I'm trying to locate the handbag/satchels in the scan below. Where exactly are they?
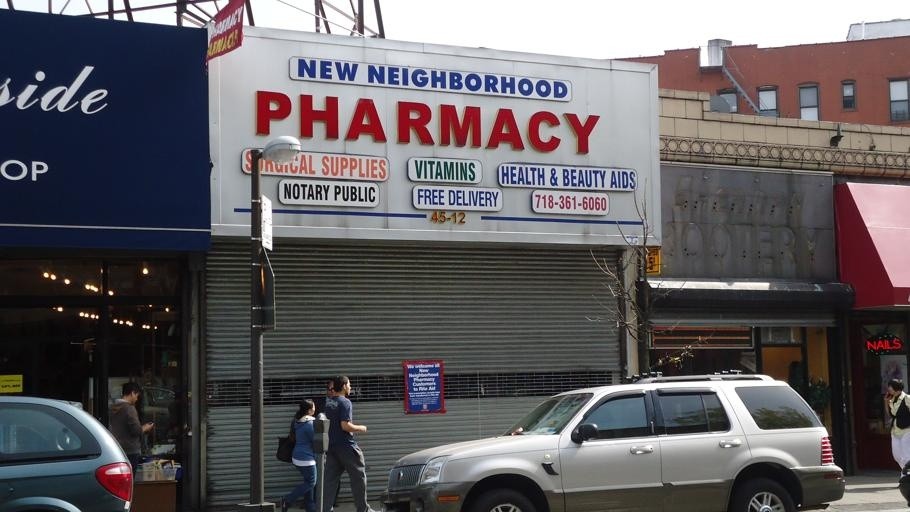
[277,437,295,463]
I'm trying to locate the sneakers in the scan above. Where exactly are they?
[280,496,289,512]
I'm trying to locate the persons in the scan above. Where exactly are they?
[883,378,910,486]
[314,379,342,508]
[106,379,156,486]
[323,375,377,512]
[279,397,318,511]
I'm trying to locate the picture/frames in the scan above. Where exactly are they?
[404,361,445,414]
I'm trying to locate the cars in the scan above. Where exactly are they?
[138,384,192,420]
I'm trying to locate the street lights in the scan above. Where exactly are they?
[235,134,304,511]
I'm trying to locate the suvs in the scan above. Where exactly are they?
[0,393,136,512]
[380,369,848,512]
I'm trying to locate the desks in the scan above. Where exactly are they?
[130,480,178,512]
